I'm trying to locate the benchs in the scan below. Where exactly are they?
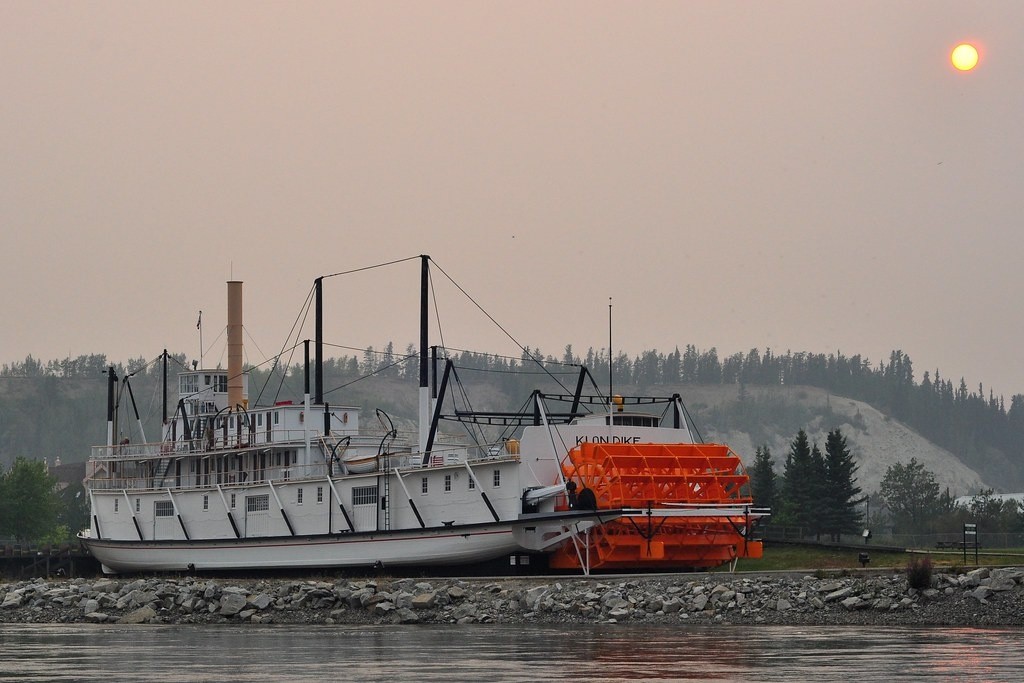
[935,545,981,548]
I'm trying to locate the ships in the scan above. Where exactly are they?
[77,251,776,578]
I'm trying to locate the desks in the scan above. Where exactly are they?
[936,541,979,550]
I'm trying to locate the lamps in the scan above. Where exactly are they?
[862,529,872,544]
[858,552,870,567]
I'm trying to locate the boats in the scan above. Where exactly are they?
[346,454,383,473]
